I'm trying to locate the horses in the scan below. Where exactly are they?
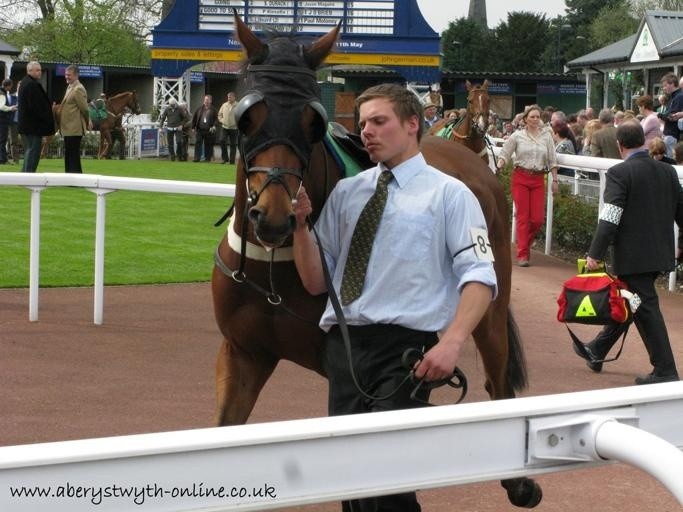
[40,89,142,160]
[427,78,490,166]
[210,5,543,509]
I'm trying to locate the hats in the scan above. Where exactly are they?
[422,100,436,109]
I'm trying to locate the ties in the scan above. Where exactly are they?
[339,168,395,307]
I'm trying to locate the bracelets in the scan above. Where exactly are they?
[552,180,557,183]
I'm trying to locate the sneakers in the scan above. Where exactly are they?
[169,156,236,164]
[516,258,530,267]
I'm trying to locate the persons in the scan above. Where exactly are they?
[18,59,56,173]
[571,118,682,385]
[158,94,216,163]
[216,92,240,166]
[0,78,22,166]
[394,72,682,202]
[290,81,498,511]
[55,64,93,189]
[496,102,558,268]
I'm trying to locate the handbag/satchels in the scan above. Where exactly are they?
[555,261,643,328]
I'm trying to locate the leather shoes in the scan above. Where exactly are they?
[571,340,603,373]
[634,370,680,385]
